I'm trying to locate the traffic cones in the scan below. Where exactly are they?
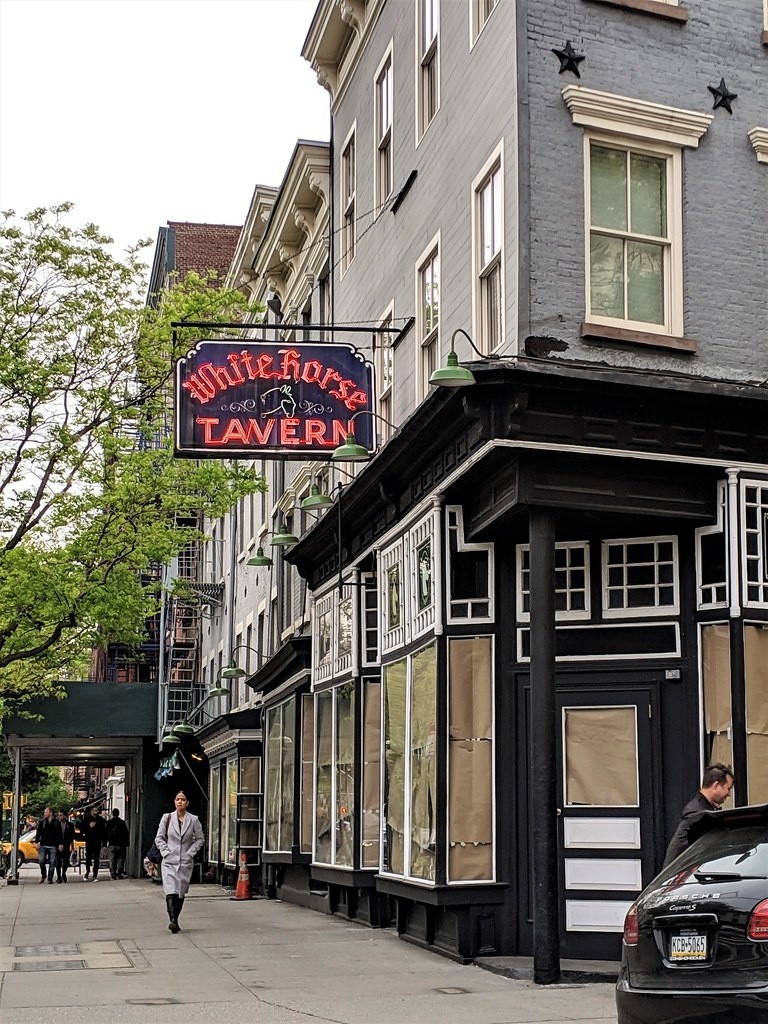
[228,855,260,900]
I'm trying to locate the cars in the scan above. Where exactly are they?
[0,828,90,868]
[613,802,768,1024]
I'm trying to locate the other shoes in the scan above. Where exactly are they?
[82,876,89,881]
[112,875,117,880]
[48,877,53,884]
[39,875,46,884]
[92,878,98,883]
[56,879,62,884]
[116,873,124,879]
[62,876,67,883]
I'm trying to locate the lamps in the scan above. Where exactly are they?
[331,410,398,460]
[429,328,491,387]
[246,532,279,565]
[269,507,319,545]
[222,645,271,679]
[301,466,354,509]
[162,720,198,743]
[173,707,216,733]
[210,666,251,696]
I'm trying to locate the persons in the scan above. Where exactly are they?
[664,762,735,867]
[102,809,130,879]
[154,791,205,934]
[36,807,60,883]
[81,807,106,882]
[27,824,35,831]
[53,809,75,883]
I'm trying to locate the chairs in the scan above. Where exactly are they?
[73,846,93,875]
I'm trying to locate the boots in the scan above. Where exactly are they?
[166,894,185,933]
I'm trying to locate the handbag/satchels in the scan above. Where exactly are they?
[148,814,172,865]
